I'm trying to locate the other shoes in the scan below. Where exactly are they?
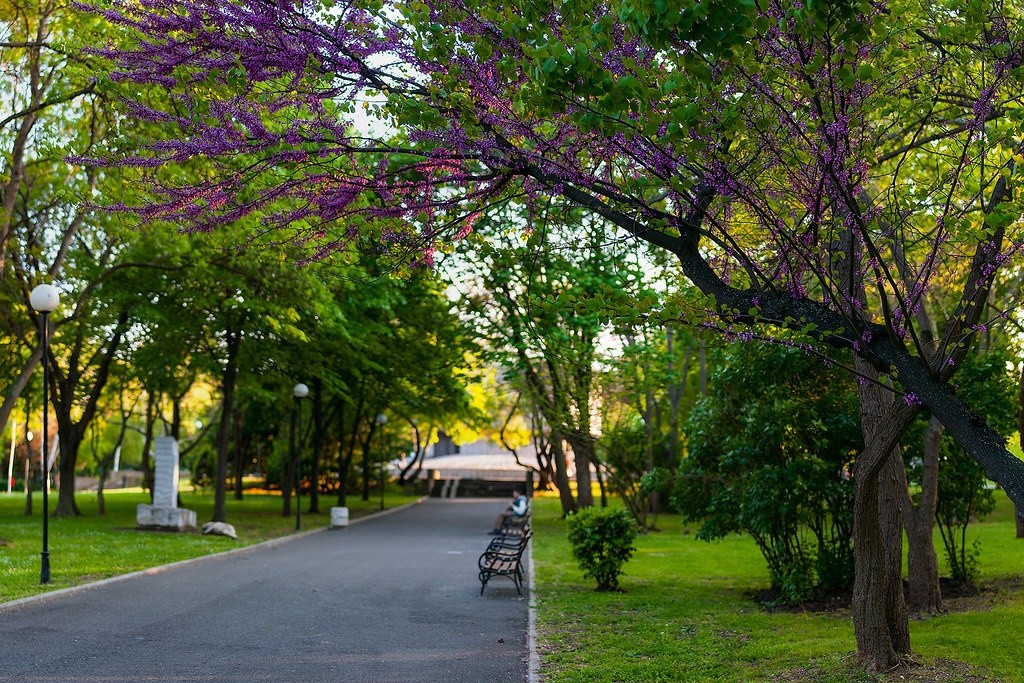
[488,528,502,535]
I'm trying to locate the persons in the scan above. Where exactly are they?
[487,486,527,535]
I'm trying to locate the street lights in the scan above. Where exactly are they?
[293,384,310,531]
[29,284,60,585]
[376,414,389,508]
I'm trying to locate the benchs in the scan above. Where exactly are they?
[477,496,534,596]
